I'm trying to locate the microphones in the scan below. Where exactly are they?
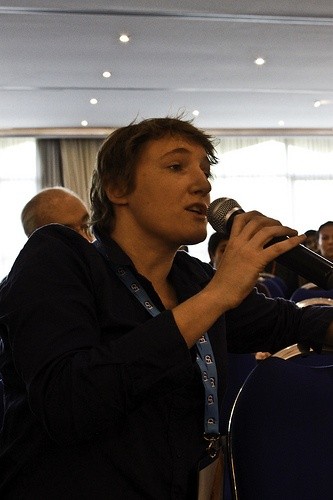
[206,198,333,293]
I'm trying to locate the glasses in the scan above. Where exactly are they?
[69,222,92,232]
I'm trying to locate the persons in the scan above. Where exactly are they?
[1,119,306,499]
[206,233,270,298]
[286,230,319,299]
[317,221,332,261]
[20,187,94,244]
